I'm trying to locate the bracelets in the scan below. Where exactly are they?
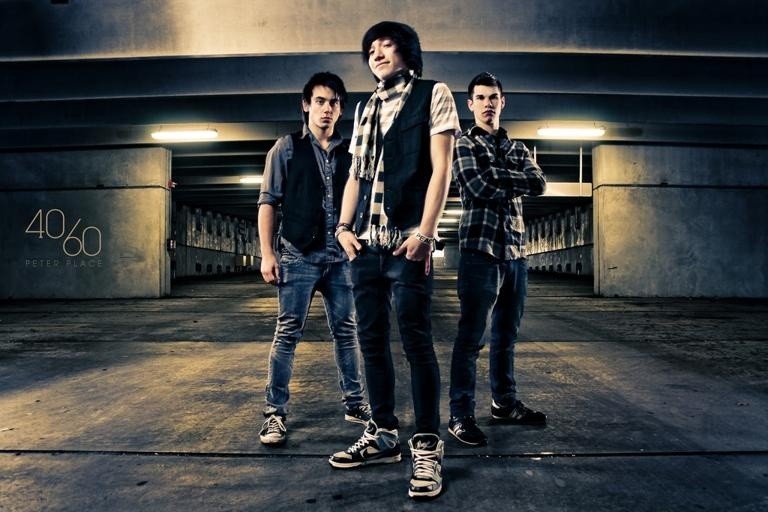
[414,232,435,247]
[334,222,352,238]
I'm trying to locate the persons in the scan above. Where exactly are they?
[324,21,464,504]
[256,70,374,448]
[445,71,550,446]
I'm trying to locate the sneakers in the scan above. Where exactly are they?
[328,417,402,469]
[490,398,548,427]
[344,400,374,427]
[258,403,288,445]
[406,432,445,499]
[447,414,488,447]
